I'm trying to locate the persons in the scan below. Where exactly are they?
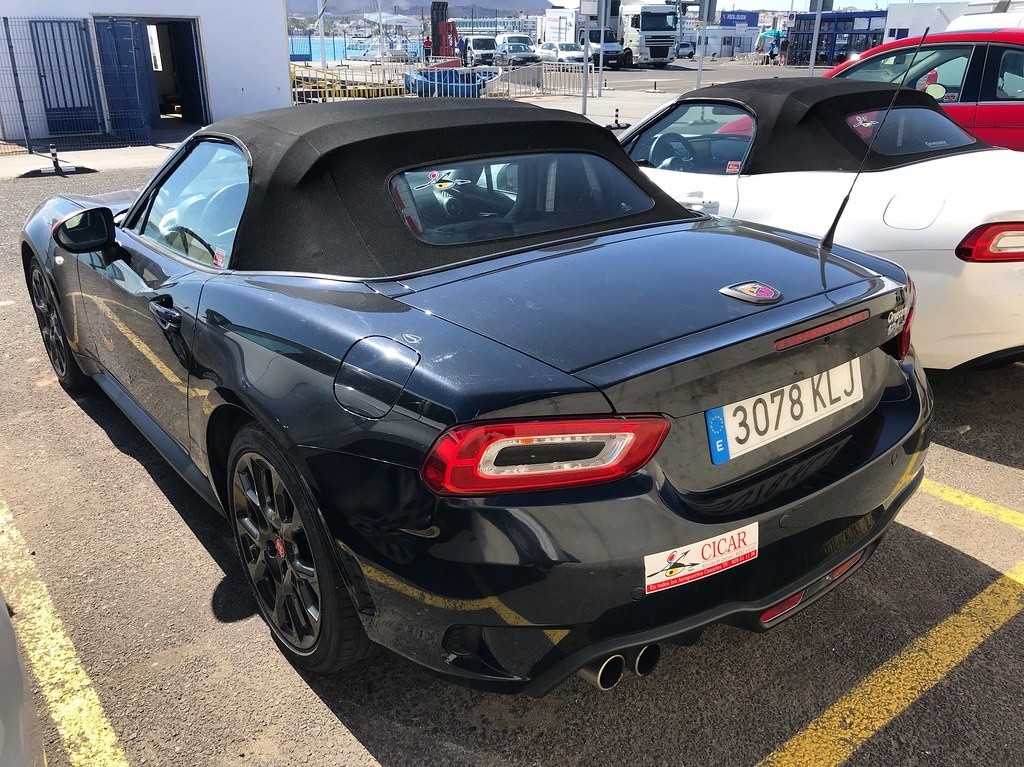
[768,33,791,66]
[422,35,433,66]
[456,35,467,70]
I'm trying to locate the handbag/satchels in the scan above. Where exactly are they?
[773,45,779,54]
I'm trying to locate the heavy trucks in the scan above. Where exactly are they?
[589,0,679,70]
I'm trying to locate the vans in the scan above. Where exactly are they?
[495,34,536,53]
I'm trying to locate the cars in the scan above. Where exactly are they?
[492,43,542,71]
[820,29,1024,153]
[536,41,594,73]
[679,42,694,58]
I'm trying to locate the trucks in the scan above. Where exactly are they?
[453,35,498,67]
[543,9,624,71]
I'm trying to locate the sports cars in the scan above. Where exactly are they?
[23,97,937,697]
[438,74,1024,375]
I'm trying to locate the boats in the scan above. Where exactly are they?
[345,35,422,62]
